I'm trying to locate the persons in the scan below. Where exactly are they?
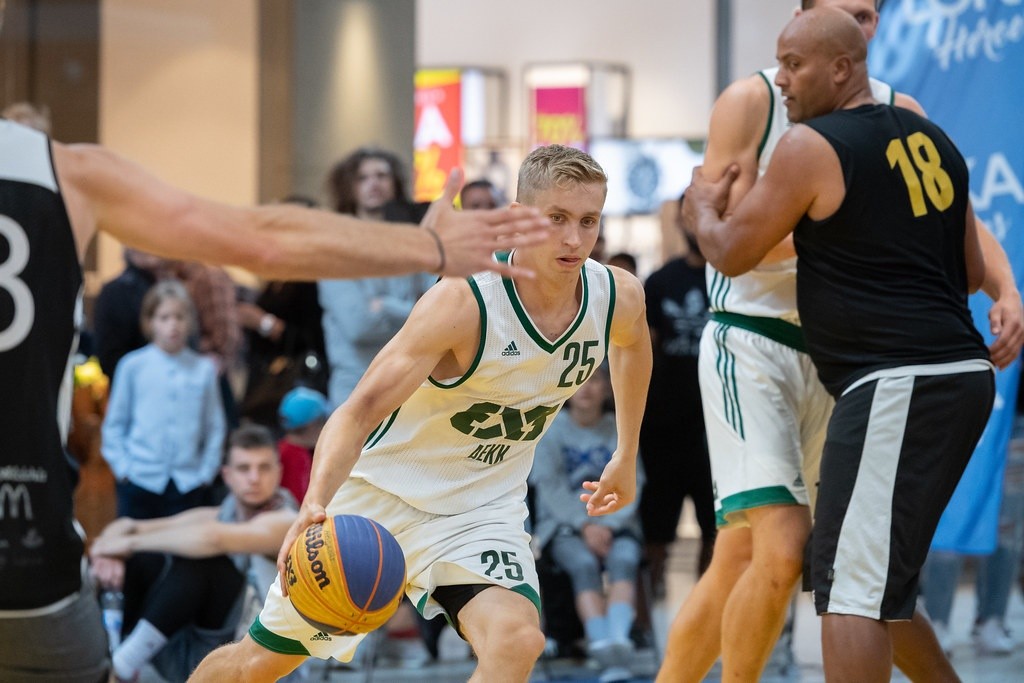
[0,0,1024,683]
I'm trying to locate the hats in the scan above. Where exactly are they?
[280,385,331,431]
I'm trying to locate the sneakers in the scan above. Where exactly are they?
[587,636,638,682]
[930,624,952,658]
[973,618,1013,656]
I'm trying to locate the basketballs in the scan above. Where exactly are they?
[282,512,410,639]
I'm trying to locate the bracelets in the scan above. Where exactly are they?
[259,313,277,335]
[425,227,446,274]
